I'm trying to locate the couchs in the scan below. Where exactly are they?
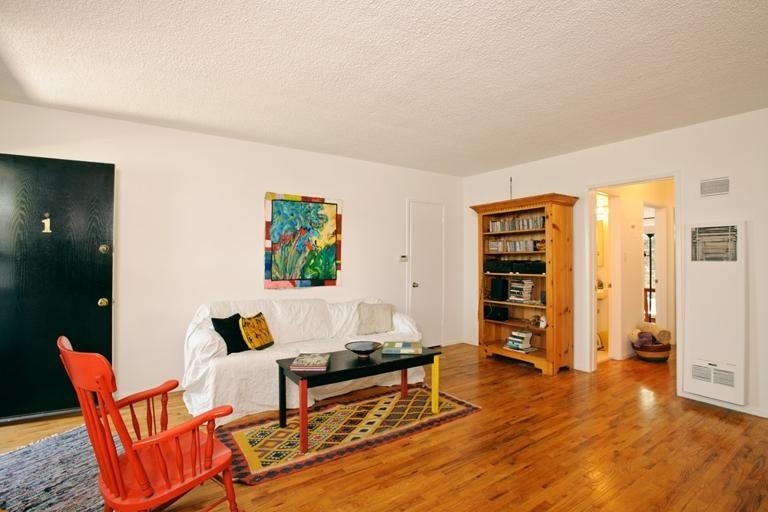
[183,298,426,431]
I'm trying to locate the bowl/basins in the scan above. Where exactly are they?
[345,341,383,358]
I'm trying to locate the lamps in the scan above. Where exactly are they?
[643,226,655,322]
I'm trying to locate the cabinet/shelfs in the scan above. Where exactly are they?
[469,191,580,376]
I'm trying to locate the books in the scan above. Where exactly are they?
[485,216,550,252]
[290,351,331,367]
[290,369,326,371]
[503,328,537,354]
[290,366,327,369]
[381,341,423,354]
[506,279,536,304]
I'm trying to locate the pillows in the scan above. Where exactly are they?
[211,313,251,356]
[359,303,392,335]
[239,312,274,351]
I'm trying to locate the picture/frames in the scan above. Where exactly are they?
[264,191,343,288]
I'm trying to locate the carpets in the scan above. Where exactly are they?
[214,385,482,486]
[0,424,126,512]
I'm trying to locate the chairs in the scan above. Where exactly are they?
[57,335,245,512]
